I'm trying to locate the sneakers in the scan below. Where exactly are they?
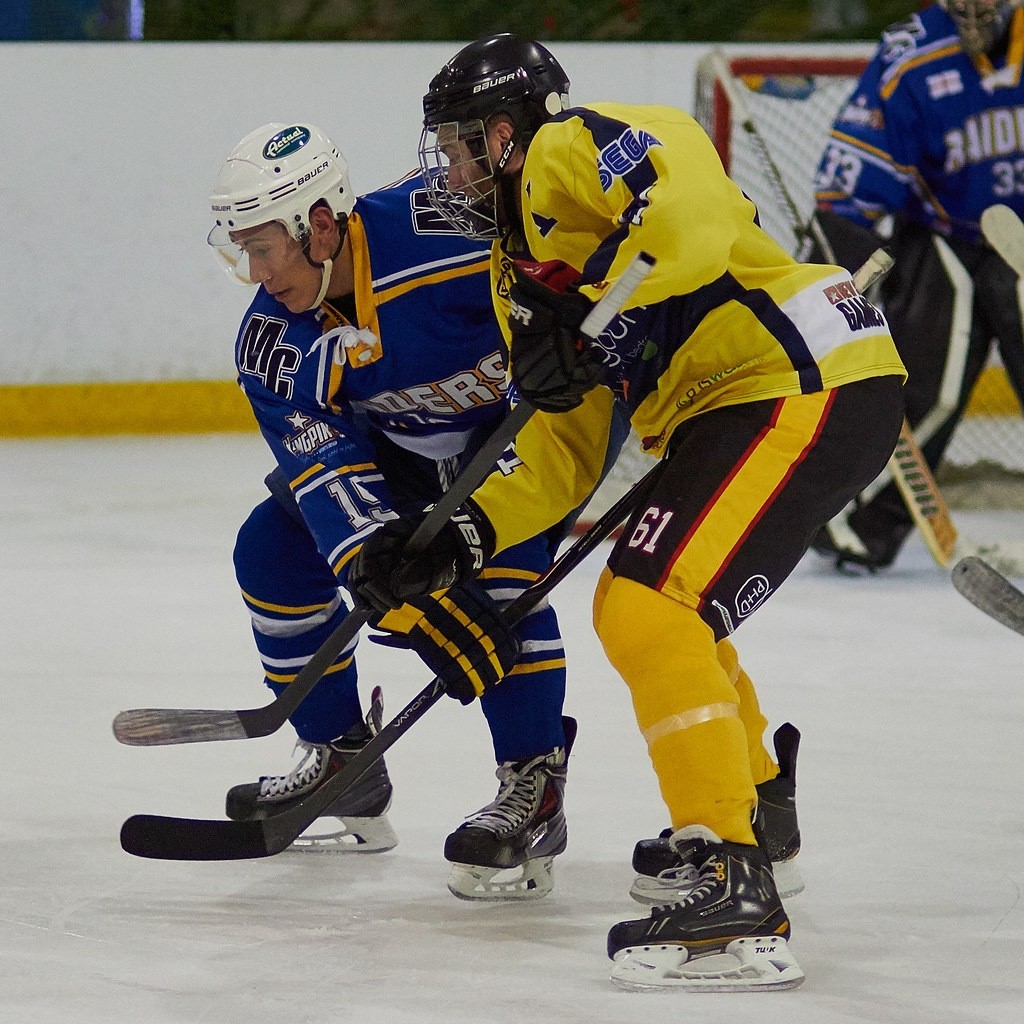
[607,797,806,993]
[443,716,577,902]
[225,686,398,855]
[630,722,806,907]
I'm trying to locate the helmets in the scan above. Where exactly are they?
[422,31,572,238]
[206,122,356,266]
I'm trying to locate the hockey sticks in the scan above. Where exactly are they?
[949,554,1024,636]
[117,248,893,865]
[702,44,1024,583]
[109,246,656,745]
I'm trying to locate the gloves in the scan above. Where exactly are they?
[508,285,603,413]
[347,496,496,614]
[366,580,522,705]
[807,208,883,274]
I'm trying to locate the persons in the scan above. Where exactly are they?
[792,1,1023,579]
[205,120,634,868]
[349,32,910,961]
